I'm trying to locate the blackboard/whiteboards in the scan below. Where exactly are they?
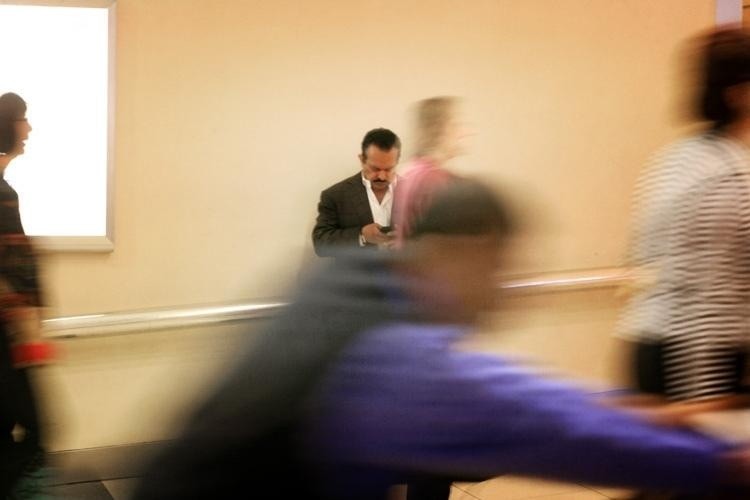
[0,0,120,253]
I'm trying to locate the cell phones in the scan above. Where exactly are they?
[379,224,393,236]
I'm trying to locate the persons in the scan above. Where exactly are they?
[389,96,463,251]
[611,22,750,430]
[121,174,749,498]
[0,90,60,497]
[311,127,401,263]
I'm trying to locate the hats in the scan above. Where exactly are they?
[401,172,519,239]
